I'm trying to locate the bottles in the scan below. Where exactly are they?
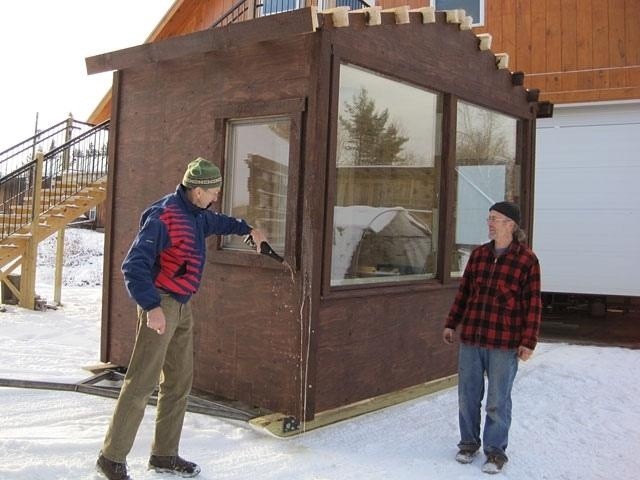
[244,233,285,264]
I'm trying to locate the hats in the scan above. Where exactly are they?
[181,156,223,190]
[488,201,521,227]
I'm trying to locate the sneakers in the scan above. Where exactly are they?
[455,447,480,467]
[482,453,507,476]
[93,455,132,480]
[146,453,202,479]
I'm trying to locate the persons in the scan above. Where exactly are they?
[441,201,542,474]
[96,157,268,480]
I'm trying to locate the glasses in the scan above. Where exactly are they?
[486,216,512,224]
